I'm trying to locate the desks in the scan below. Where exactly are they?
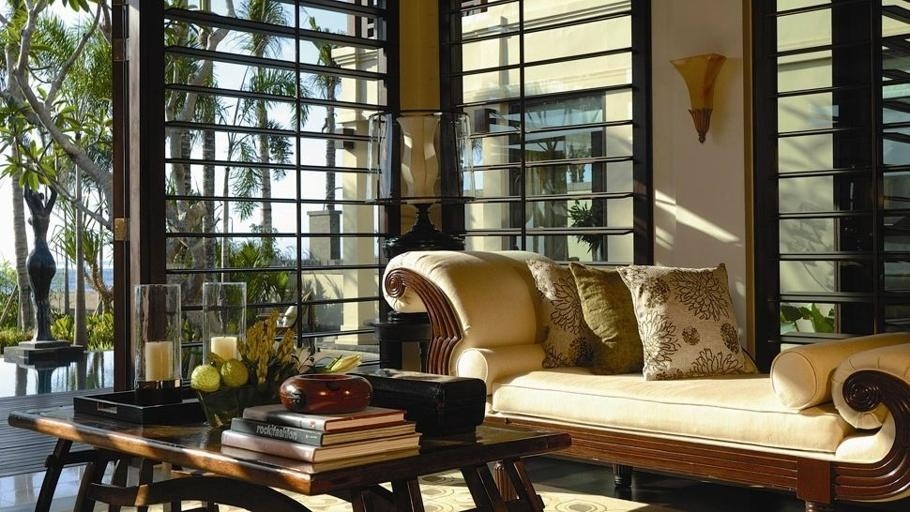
[9,406,571,511]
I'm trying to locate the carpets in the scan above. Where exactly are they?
[92,468,681,512]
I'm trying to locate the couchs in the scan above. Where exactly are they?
[381,250,910,512]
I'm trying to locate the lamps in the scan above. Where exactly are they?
[366,109,476,323]
[669,53,726,143]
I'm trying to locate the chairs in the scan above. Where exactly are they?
[256,292,312,332]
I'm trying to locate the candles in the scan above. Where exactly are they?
[145,341,172,382]
[211,336,235,360]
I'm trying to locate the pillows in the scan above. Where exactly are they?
[525,258,759,381]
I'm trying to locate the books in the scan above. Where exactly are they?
[243,405,406,431]
[220,429,424,464]
[231,417,417,446]
